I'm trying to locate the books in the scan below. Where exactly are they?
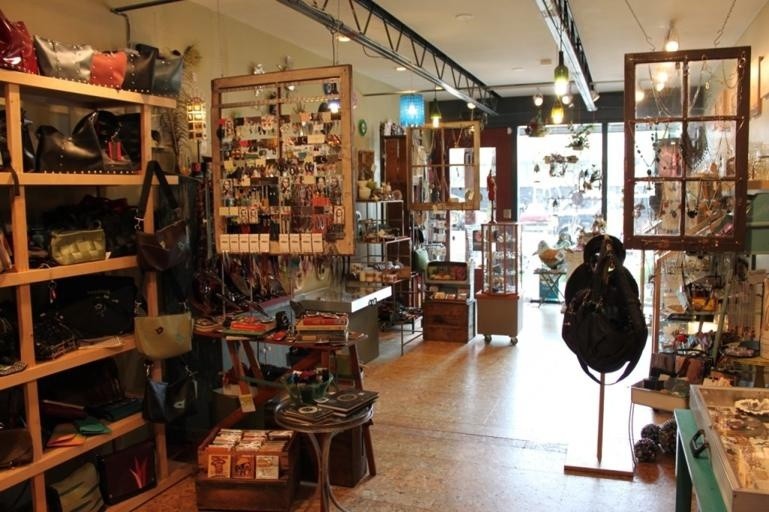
[316,386,379,413]
[279,402,336,421]
[219,311,351,346]
[206,428,295,479]
[332,399,377,418]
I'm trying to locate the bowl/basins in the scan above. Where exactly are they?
[281,371,334,406]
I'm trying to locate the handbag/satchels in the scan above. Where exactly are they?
[133,218,193,273]
[50,462,106,512]
[142,369,209,424]
[0,195,138,273]
[0,359,143,470]
[563,287,648,374]
[0,275,148,376]
[0,107,135,174]
[133,313,194,360]
[649,351,712,397]
[100,439,157,505]
[0,10,186,95]
[414,249,430,273]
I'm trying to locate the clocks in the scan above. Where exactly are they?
[359,120,368,136]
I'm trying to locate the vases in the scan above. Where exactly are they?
[357,180,371,200]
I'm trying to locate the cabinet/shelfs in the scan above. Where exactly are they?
[381,135,429,324]
[638,197,749,413]
[421,261,476,344]
[0,68,191,512]
[475,290,518,345]
[481,223,519,296]
[338,200,416,333]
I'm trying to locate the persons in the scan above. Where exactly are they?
[249,209,258,224]
[304,161,310,176]
[330,177,340,191]
[316,177,326,192]
[308,162,314,176]
[335,208,344,224]
[221,179,233,198]
[241,209,248,224]
[280,176,291,194]
[289,167,296,176]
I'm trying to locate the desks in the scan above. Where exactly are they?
[195,329,379,479]
[670,407,727,512]
[533,269,567,308]
[273,394,372,512]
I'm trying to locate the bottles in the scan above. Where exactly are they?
[754,156,768,180]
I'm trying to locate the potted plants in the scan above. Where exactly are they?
[564,120,592,150]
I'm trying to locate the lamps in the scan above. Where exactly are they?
[550,100,565,125]
[190,97,207,163]
[553,51,569,98]
[362,78,643,108]
[429,98,443,129]
[327,89,341,114]
[398,94,427,128]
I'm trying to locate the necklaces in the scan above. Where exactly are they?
[627,117,737,228]
